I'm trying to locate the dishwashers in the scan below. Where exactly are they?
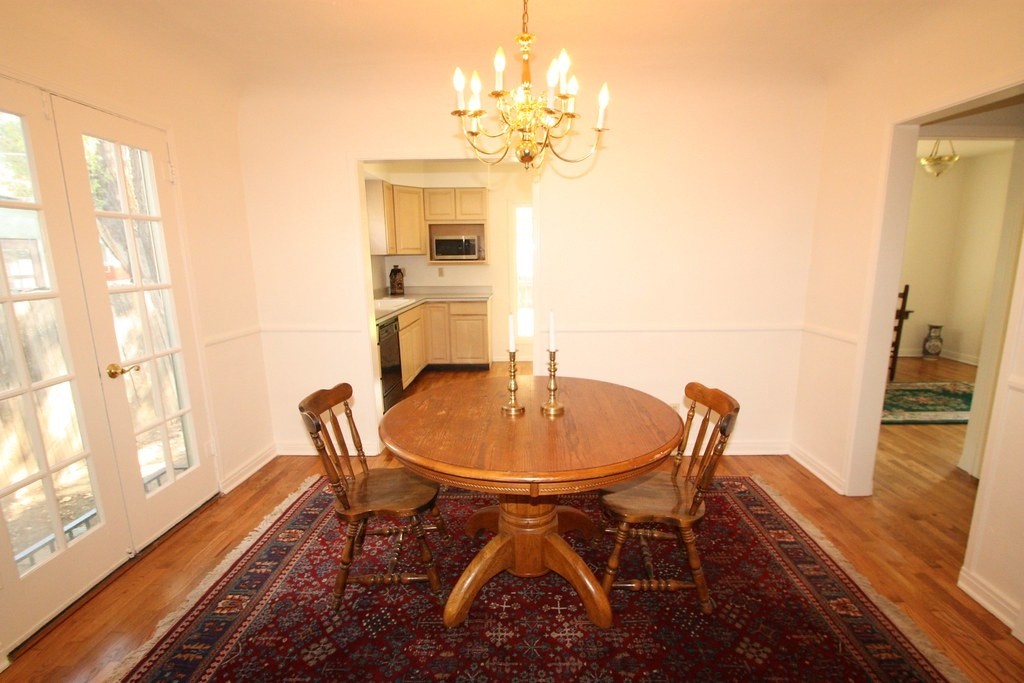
[378,318,404,414]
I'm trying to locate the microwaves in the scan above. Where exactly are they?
[433,235,481,260]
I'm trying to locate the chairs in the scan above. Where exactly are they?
[299,382,444,602]
[597,382,739,614]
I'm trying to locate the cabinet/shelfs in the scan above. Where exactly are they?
[381,181,396,255]
[426,301,490,366]
[423,186,487,225]
[398,304,429,390]
[392,184,428,256]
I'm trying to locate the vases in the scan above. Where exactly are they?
[922,323,944,362]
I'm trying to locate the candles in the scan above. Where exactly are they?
[539,310,565,416]
[500,313,527,416]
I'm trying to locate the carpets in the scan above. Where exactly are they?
[880,381,975,425]
[106,470,971,683]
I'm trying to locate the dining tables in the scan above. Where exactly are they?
[375,374,686,631]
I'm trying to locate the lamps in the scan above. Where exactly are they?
[918,138,962,179]
[449,0,612,181]
